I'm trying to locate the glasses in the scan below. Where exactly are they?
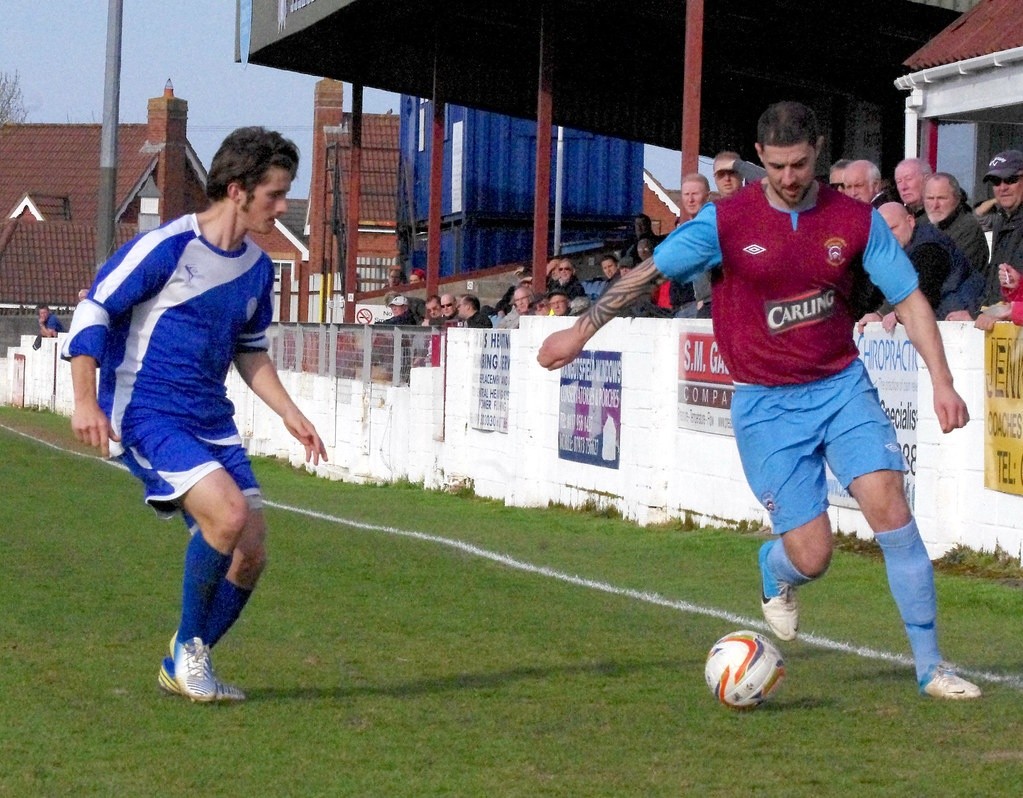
[829,183,845,191]
[558,268,570,271]
[990,174,1023,186]
[440,303,452,308]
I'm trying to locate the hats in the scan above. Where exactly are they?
[619,255,634,267]
[409,268,426,279]
[983,149,1023,183]
[389,296,408,306]
[520,276,533,283]
[514,266,527,275]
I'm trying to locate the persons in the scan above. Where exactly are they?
[59,125,328,703]
[830,160,904,213]
[894,158,931,225]
[970,198,999,232]
[78,289,90,301]
[38,305,65,338]
[692,151,767,319]
[974,263,1023,330]
[857,202,986,334]
[409,269,426,285]
[382,265,407,288]
[982,150,1023,303]
[536,101,982,699]
[371,153,712,381]
[915,172,989,275]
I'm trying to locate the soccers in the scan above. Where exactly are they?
[703,629,787,711]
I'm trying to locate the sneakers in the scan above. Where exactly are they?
[158,658,246,701]
[924,666,981,699]
[170,630,219,703]
[759,540,798,642]
